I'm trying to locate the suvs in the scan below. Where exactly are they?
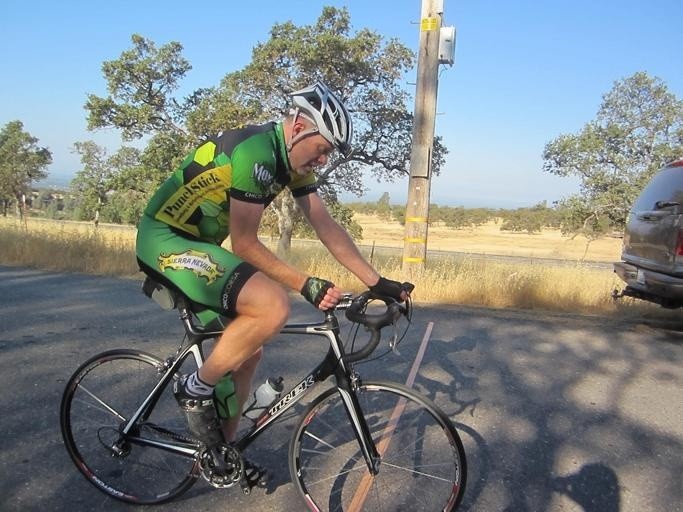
[610,157,682,311]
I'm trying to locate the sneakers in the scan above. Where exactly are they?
[244,459,272,482]
[172,373,223,442]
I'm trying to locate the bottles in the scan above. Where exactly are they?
[242,375,284,420]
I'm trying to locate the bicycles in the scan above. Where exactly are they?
[57,269,468,511]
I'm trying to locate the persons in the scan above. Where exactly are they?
[136,80,410,483]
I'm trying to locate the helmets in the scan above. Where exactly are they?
[288,80,353,160]
